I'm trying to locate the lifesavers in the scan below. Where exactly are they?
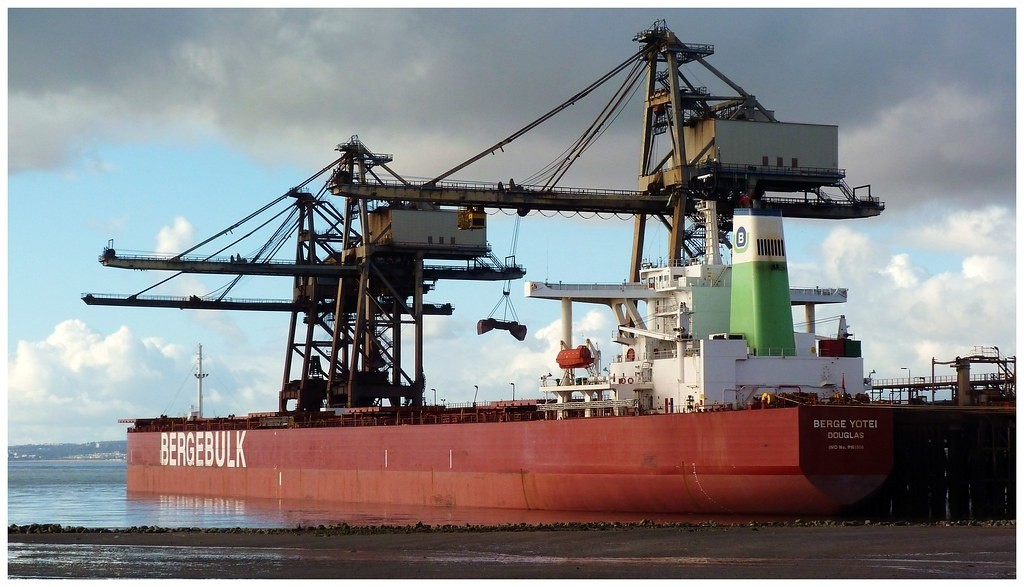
[620,377,626,384]
[761,392,771,404]
[628,377,633,384]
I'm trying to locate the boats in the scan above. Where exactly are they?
[81,18,894,516]
